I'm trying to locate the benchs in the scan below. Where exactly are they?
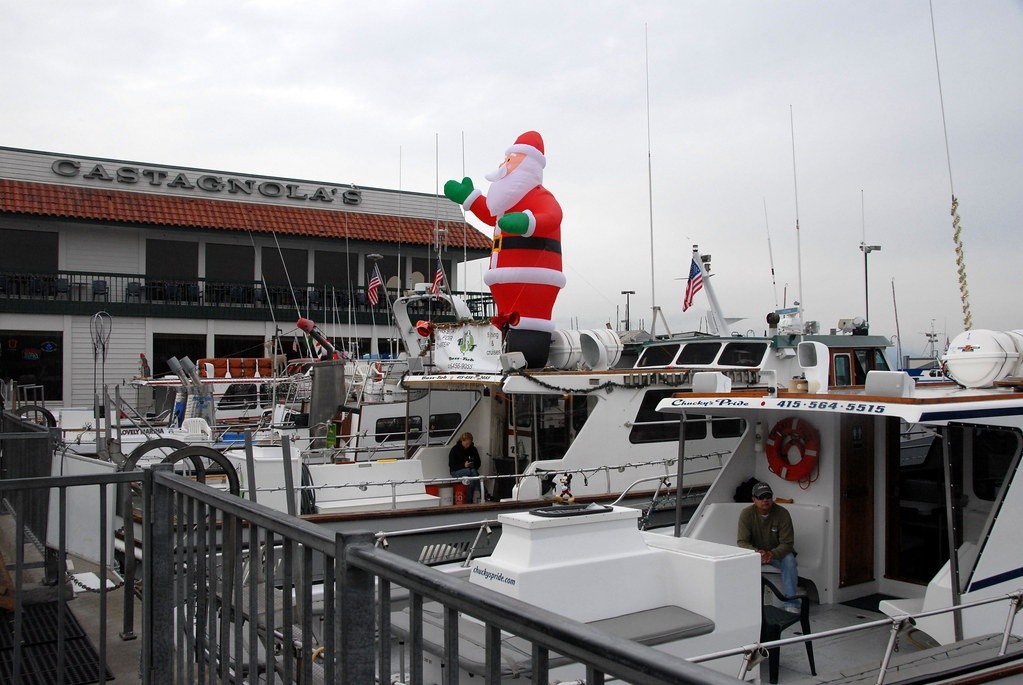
[875,544,977,685]
[412,447,491,486]
[380,595,716,685]
[820,594,1021,685]
[692,499,832,577]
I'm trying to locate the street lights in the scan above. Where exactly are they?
[859,241,883,333]
[621,290,638,333]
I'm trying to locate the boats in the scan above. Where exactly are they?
[0,131,1023,685]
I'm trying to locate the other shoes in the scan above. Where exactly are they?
[480,492,492,501]
[785,605,800,613]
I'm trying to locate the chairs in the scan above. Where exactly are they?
[761,576,815,685]
[53,278,71,300]
[182,417,212,441]
[91,280,109,303]
[126,282,143,303]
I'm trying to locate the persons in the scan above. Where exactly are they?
[737,482,800,615]
[444,131,566,369]
[448,432,493,504]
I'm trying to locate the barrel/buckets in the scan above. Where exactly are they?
[425,483,467,506]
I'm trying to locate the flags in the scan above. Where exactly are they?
[367,267,381,305]
[682,258,703,312]
[431,259,443,299]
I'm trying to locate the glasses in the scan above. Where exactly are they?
[755,493,772,500]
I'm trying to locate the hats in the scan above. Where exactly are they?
[751,482,772,497]
